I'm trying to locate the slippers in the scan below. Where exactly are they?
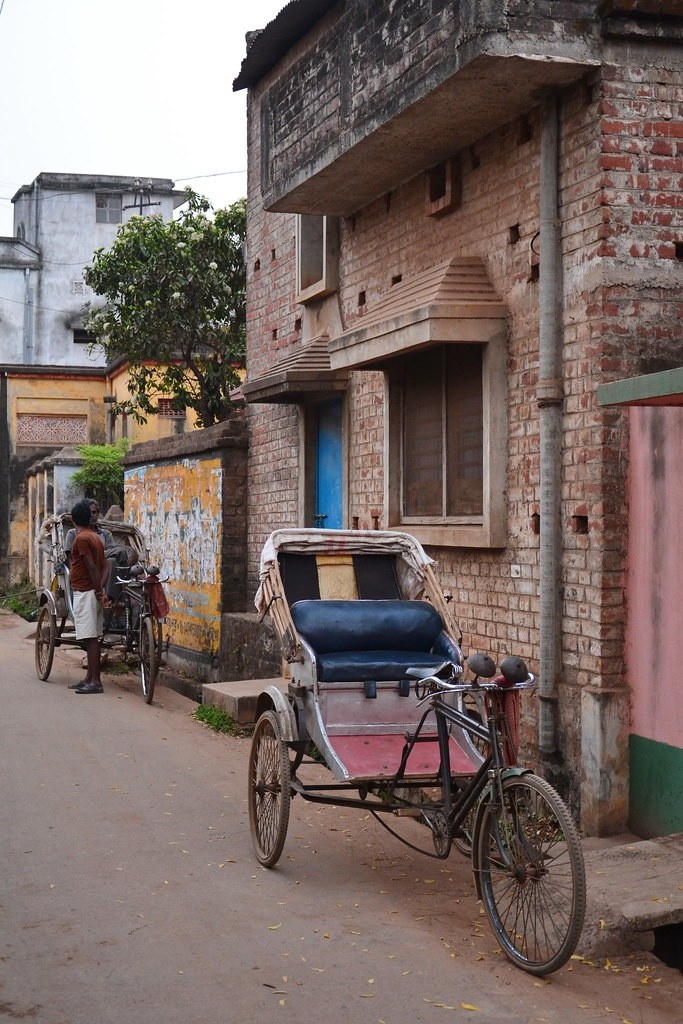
[67,679,88,689]
[75,684,104,694]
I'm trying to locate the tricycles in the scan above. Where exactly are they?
[36,510,171,704]
[248,527,587,974]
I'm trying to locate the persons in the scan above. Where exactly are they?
[66,504,112,693]
[64,499,132,609]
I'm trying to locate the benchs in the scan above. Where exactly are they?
[290,599,455,684]
[57,565,144,597]
